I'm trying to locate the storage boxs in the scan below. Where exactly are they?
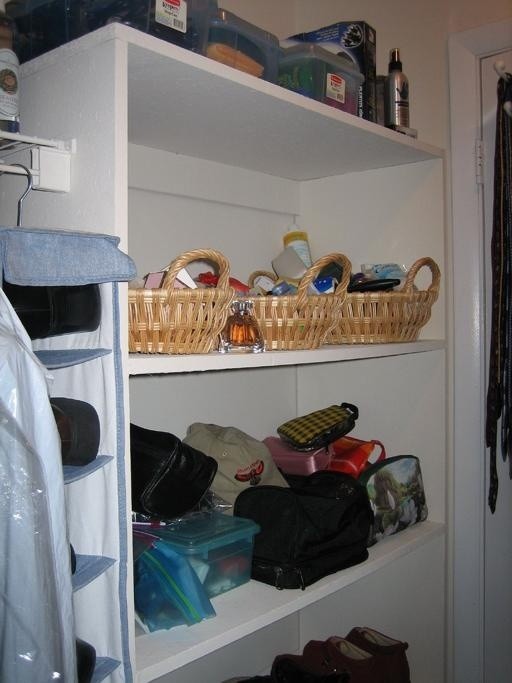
[5,0,218,66]
[287,19,377,124]
[207,8,280,82]
[280,44,361,117]
[133,511,261,598]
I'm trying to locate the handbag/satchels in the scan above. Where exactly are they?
[262,437,336,476]
[277,403,358,452]
[130,422,218,520]
[326,436,386,480]
[182,422,291,516]
[233,470,375,590]
[357,455,428,548]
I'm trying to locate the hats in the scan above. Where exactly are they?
[49,398,100,466]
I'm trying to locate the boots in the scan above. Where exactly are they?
[303,636,375,682]
[345,627,410,683]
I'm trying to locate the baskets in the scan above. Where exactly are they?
[218,254,351,351]
[128,248,235,355]
[248,257,441,345]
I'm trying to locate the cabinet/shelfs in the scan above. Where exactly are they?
[0,129,136,681]
[13,24,450,682]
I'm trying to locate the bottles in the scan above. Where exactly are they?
[283,224,313,271]
[0,15,22,135]
[384,47,410,135]
[219,301,265,353]
[375,75,388,127]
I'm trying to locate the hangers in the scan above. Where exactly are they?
[0,161,33,227]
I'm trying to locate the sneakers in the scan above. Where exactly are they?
[233,654,352,683]
